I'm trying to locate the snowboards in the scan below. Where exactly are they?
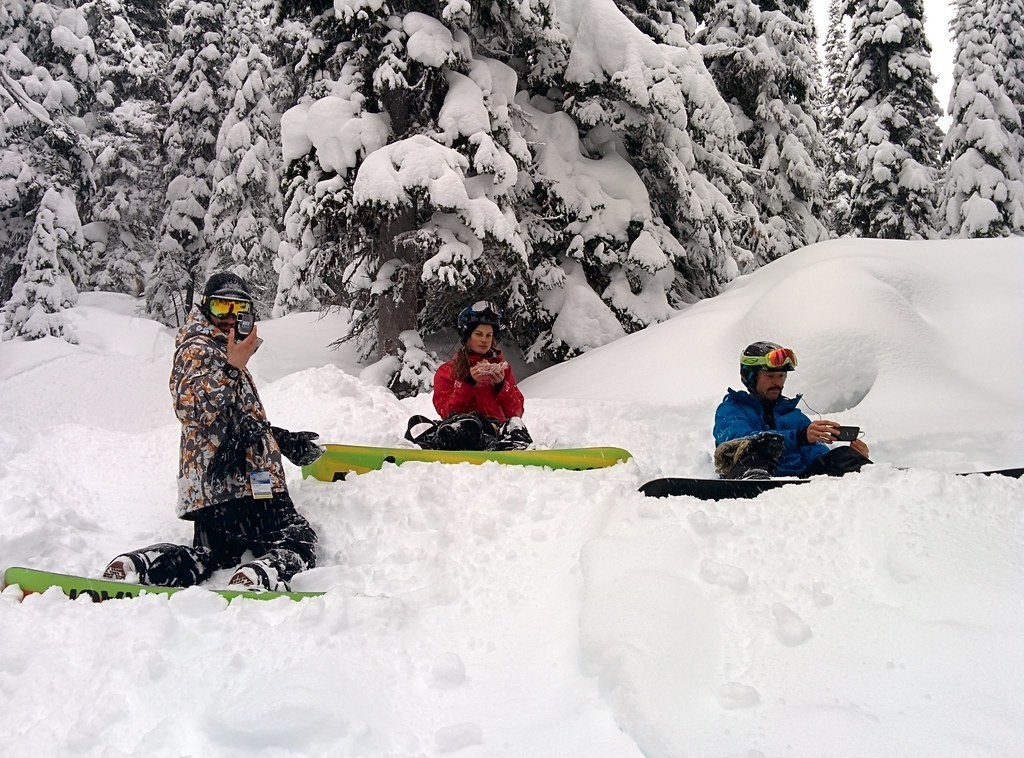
[637,466,1024,502]
[4,565,329,604]
[300,442,632,484]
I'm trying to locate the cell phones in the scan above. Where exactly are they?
[831,426,860,441]
[234,311,256,341]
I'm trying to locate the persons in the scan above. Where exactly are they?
[713,341,874,481]
[431,302,532,451]
[102,271,324,593]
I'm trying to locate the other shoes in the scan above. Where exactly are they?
[104,544,217,587]
[229,541,316,592]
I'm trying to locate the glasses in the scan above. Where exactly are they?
[742,349,796,370]
[465,300,503,319]
[206,296,254,321]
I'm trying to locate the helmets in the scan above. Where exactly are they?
[742,343,795,391]
[460,300,501,346]
[201,272,255,329]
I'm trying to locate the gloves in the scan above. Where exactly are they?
[270,426,325,468]
